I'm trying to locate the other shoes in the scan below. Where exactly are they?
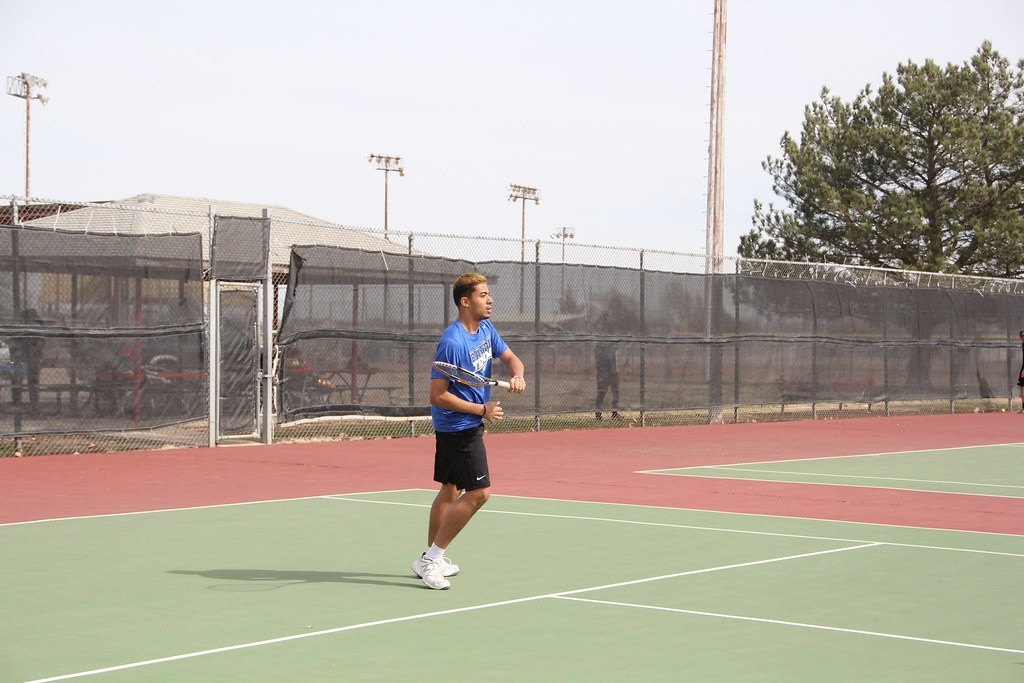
[611,411,625,421]
[595,411,603,421]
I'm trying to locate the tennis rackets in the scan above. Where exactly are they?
[431,360,511,391]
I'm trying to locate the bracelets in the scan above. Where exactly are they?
[512,375,524,380]
[481,404,486,417]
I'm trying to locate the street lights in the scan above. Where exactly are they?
[368,153,407,236]
[550,226,576,310]
[507,184,540,313]
[5,71,50,203]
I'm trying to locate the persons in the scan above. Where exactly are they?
[410,272,527,590]
[1017,330,1024,412]
[22,308,46,405]
[593,327,623,420]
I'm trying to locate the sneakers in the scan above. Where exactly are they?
[411,552,460,590]
